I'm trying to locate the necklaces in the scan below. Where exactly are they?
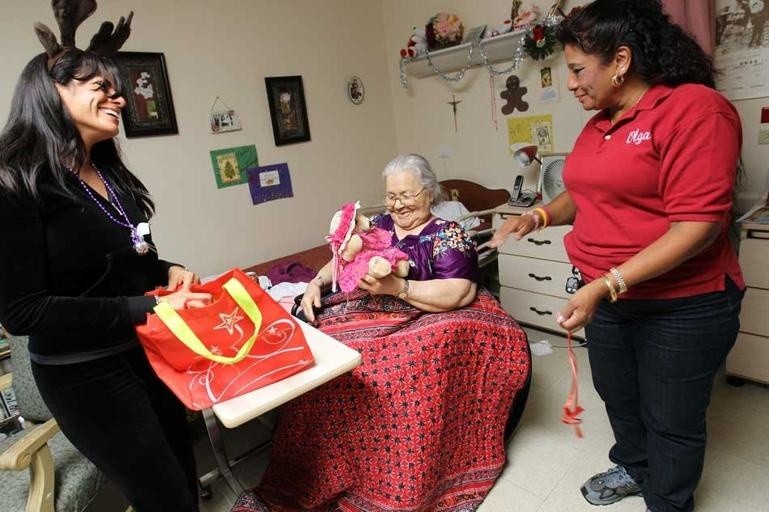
[62,159,149,255]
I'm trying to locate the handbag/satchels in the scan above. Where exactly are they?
[134,268,315,411]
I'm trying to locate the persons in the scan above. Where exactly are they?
[1,1,216,511]
[300,152,482,324]
[484,1,748,512]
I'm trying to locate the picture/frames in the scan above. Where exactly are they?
[208,111,242,133]
[111,51,178,138]
[266,75,310,146]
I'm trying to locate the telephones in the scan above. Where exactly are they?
[507,175,538,208]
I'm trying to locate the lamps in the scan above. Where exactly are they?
[514,145,541,168]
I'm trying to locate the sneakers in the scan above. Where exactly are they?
[582,465,647,506]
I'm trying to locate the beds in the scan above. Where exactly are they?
[439,179,511,285]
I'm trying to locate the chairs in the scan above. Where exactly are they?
[1,337,131,511]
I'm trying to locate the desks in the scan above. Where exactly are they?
[196,316,362,503]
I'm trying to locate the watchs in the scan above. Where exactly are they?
[397,278,409,300]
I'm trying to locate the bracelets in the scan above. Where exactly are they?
[519,211,540,233]
[532,205,552,228]
[609,266,629,296]
[599,273,619,304]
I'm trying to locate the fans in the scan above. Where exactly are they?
[540,157,570,205]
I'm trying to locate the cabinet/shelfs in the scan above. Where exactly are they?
[492,198,586,342]
[725,224,769,386]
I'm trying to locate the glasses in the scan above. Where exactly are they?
[381,187,426,207]
